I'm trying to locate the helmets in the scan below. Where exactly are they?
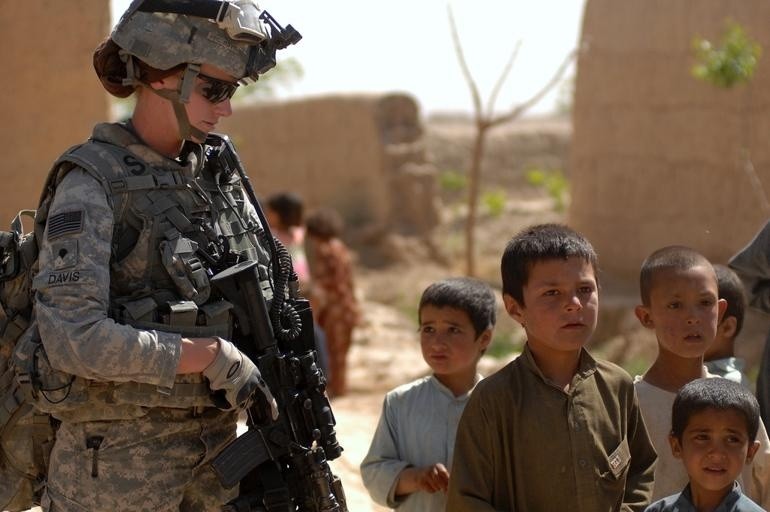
[111,0,252,80]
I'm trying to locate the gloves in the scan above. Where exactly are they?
[201,335,280,423]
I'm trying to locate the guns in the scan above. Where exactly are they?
[201,259,346,511]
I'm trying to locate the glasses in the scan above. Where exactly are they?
[197,73,241,104]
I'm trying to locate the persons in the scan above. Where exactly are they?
[645,376,769,512]
[299,207,371,397]
[625,244,768,512]
[26,0,302,510]
[257,189,309,304]
[443,221,661,509]
[359,274,498,511]
[703,264,757,391]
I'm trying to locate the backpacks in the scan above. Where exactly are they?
[0,141,138,511]
